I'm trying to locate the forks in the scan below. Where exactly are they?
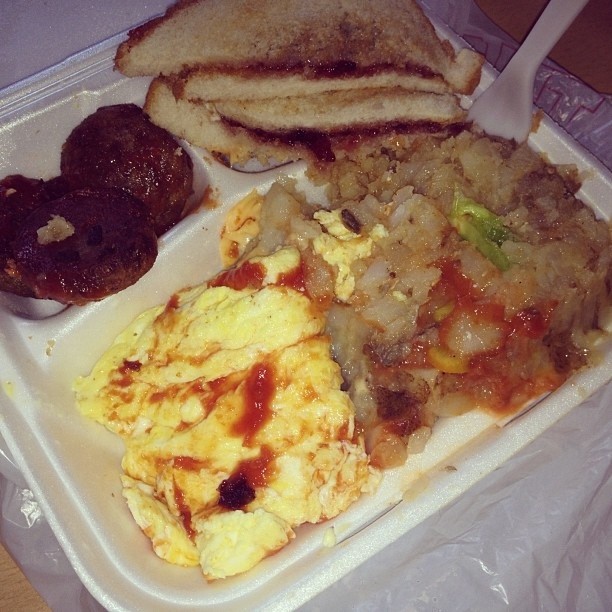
[469,0,589,146]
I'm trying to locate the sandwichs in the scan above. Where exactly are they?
[111,0,484,171]
